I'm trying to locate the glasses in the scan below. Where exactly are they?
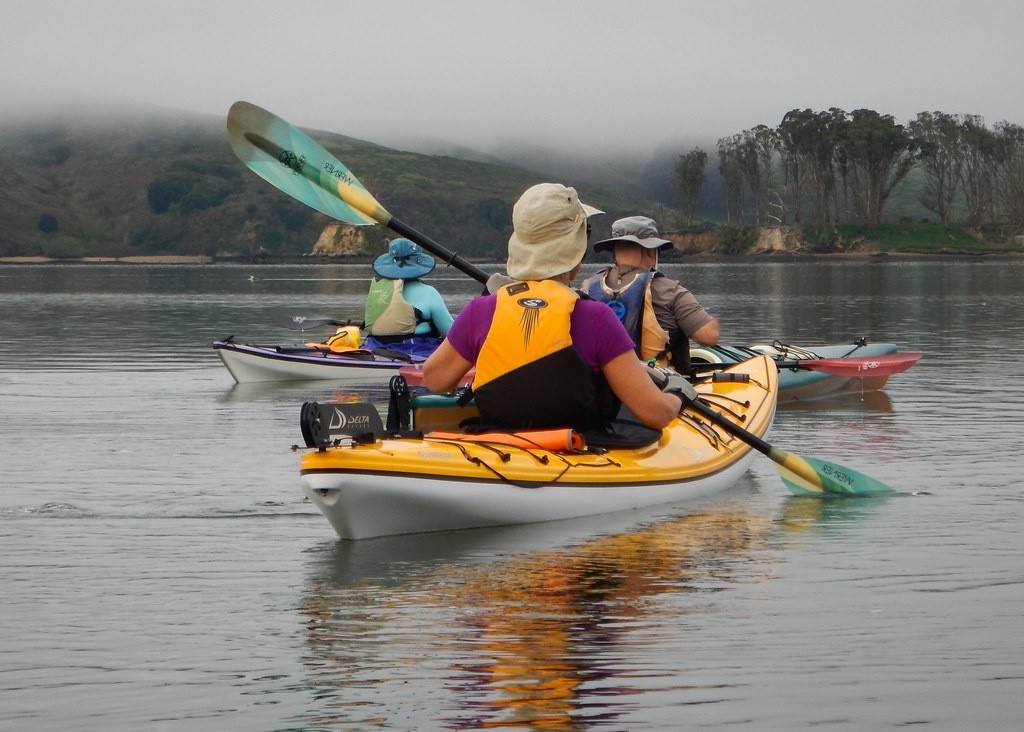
[586,224,592,240]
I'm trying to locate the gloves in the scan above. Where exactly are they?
[661,373,699,414]
[481,273,516,296]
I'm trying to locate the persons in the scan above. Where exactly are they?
[423,183,698,434]
[579,215,720,384]
[364,238,455,360]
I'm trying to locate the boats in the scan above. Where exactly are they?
[400,342,898,432]
[212,317,448,387]
[294,355,779,542]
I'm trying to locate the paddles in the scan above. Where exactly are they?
[276,342,373,353]
[225,100,901,496]
[690,352,924,377]
[270,306,365,330]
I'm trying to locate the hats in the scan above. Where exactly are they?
[373,237,436,279]
[507,183,607,281]
[593,215,675,254]
